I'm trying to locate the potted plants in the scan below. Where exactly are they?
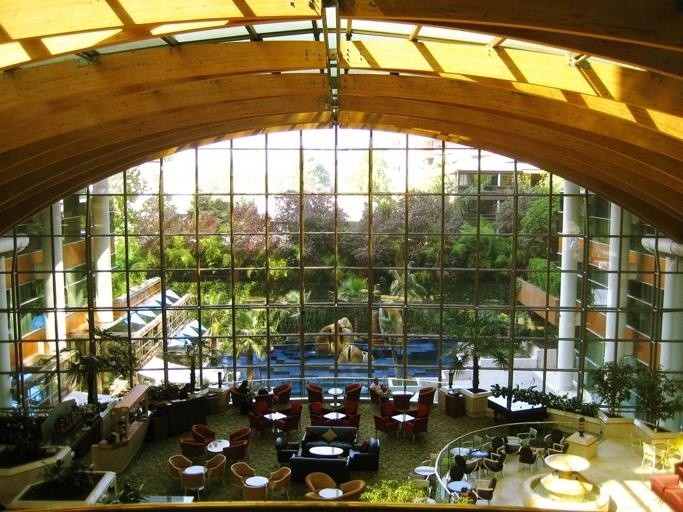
[431,303,682,459]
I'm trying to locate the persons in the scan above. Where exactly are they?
[179,383,188,400]
[238,379,253,401]
[369,378,391,402]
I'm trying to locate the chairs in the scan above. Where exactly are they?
[165,377,437,504]
[627,427,683,472]
[406,421,570,506]
